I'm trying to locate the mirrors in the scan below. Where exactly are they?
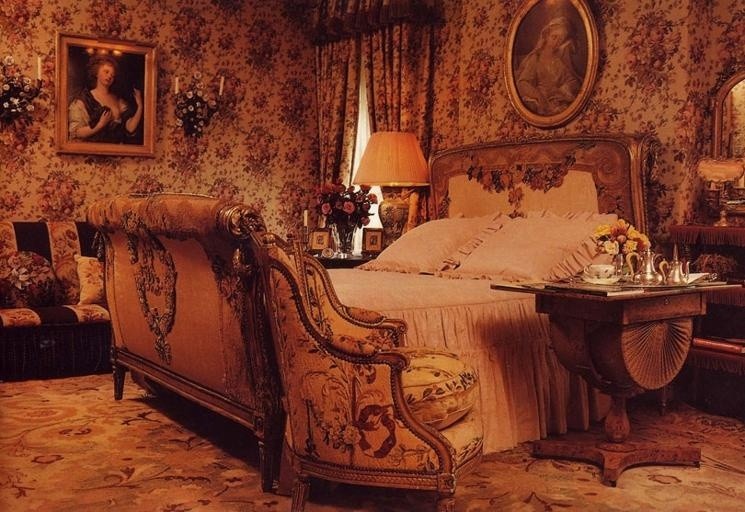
[713,71,745,204]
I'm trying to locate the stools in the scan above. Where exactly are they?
[691,335,744,423]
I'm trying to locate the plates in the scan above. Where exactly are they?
[620,285,691,291]
[581,276,620,284]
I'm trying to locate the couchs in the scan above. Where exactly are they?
[1,219,110,378]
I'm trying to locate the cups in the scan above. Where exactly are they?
[583,265,615,280]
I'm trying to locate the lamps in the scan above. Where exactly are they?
[353,131,427,250]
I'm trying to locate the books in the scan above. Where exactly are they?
[544,283,644,296]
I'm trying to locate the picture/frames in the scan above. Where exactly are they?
[54,30,155,157]
[309,227,332,254]
[503,0,598,128]
[362,227,382,254]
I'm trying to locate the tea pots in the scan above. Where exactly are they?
[626,248,662,286]
[659,255,690,286]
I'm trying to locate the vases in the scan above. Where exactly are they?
[337,225,357,256]
[616,255,637,276]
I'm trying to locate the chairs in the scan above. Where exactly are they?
[261,231,484,512]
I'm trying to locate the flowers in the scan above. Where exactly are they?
[315,177,379,228]
[170,74,228,140]
[1,55,50,125]
[589,218,651,255]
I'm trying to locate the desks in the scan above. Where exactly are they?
[672,222,744,333]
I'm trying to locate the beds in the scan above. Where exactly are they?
[108,134,656,486]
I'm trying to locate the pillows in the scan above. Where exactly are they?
[72,250,105,307]
[0,252,69,303]
[439,210,619,289]
[355,210,503,275]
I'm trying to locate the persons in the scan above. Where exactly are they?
[68,54,143,143]
